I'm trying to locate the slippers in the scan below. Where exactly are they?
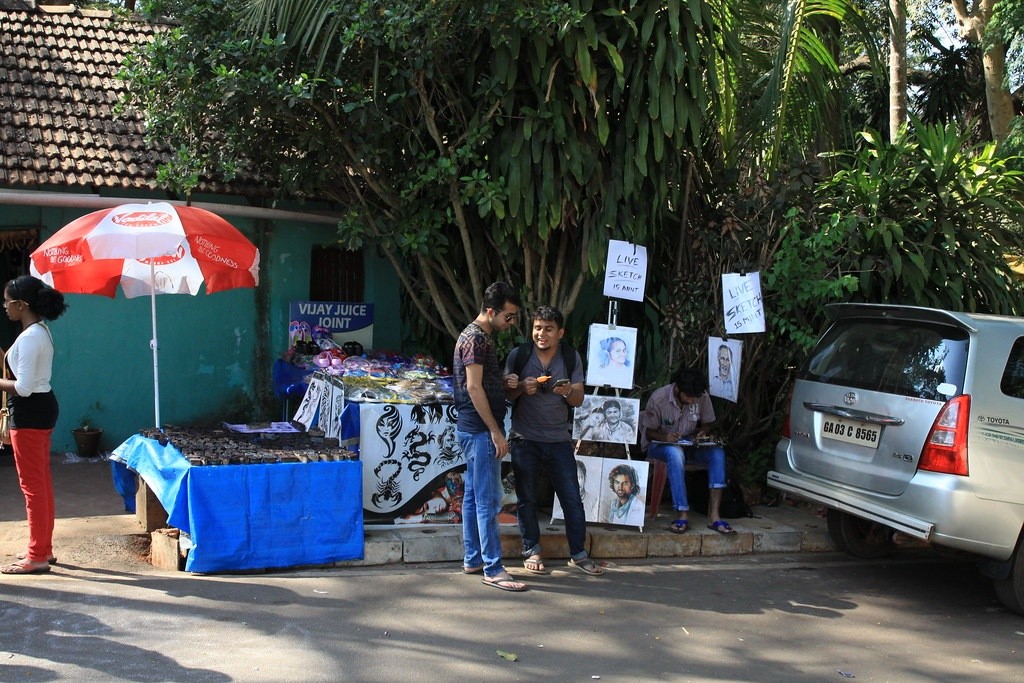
[0,562,51,574]
[17,552,57,564]
[481,577,529,591]
[461,565,483,575]
[567,560,604,576]
[523,558,546,575]
[708,520,738,536]
[670,519,691,533]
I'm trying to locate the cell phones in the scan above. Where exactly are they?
[552,379,570,388]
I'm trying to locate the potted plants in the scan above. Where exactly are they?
[70,399,103,456]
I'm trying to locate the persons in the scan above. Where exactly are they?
[0,276,66,574]
[503,305,603,576]
[453,282,528,591]
[641,367,736,534]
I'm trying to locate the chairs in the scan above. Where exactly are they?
[643,456,708,522]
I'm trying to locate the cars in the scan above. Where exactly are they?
[765,303,1024,617]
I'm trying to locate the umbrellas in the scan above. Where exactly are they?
[28,200,261,428]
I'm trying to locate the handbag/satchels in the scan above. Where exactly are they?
[0,407,11,445]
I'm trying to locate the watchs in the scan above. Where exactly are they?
[562,388,574,399]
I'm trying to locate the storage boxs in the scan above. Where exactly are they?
[136,476,169,532]
[150,532,189,571]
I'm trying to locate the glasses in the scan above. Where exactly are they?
[2,300,29,308]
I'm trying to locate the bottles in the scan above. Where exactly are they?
[11,259,16,275]
